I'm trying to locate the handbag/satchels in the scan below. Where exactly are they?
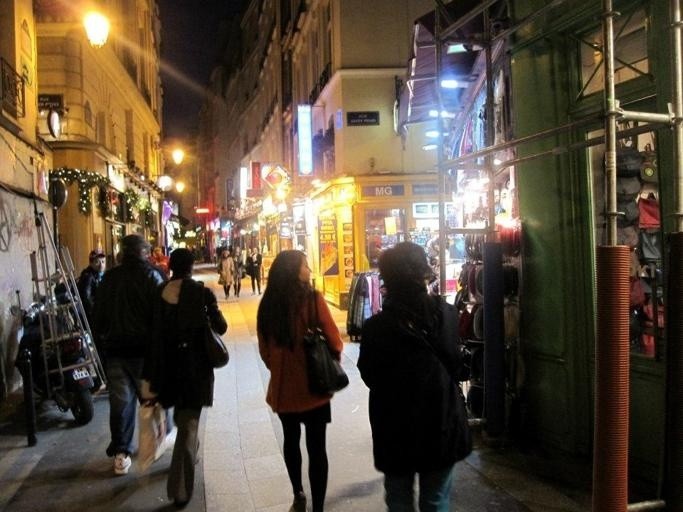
[202,322,229,367]
[305,323,348,396]
[617,143,663,356]
[454,385,469,432]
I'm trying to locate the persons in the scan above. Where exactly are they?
[148,247,170,283]
[355,239,474,512]
[77,247,111,325]
[217,248,234,299]
[245,247,263,296]
[132,246,229,511]
[230,244,245,299]
[89,234,180,477]
[255,247,342,511]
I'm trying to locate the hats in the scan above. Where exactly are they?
[119,235,150,251]
[88,248,106,261]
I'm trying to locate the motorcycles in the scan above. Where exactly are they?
[8,289,95,425]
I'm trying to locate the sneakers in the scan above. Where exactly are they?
[293,488,307,512]
[114,455,132,475]
